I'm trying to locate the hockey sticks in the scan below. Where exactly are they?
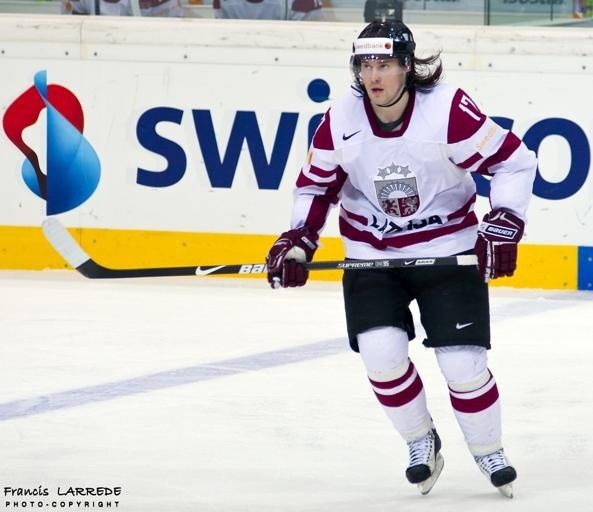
[39,218,480,279]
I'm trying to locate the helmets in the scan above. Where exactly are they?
[350,19,414,78]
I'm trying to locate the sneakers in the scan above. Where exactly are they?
[474,450,517,488]
[406,428,441,483]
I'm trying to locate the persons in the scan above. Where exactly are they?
[262,14,543,490]
[62,0,323,21]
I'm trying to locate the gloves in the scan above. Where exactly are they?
[475,208,526,281]
[266,224,317,289]
